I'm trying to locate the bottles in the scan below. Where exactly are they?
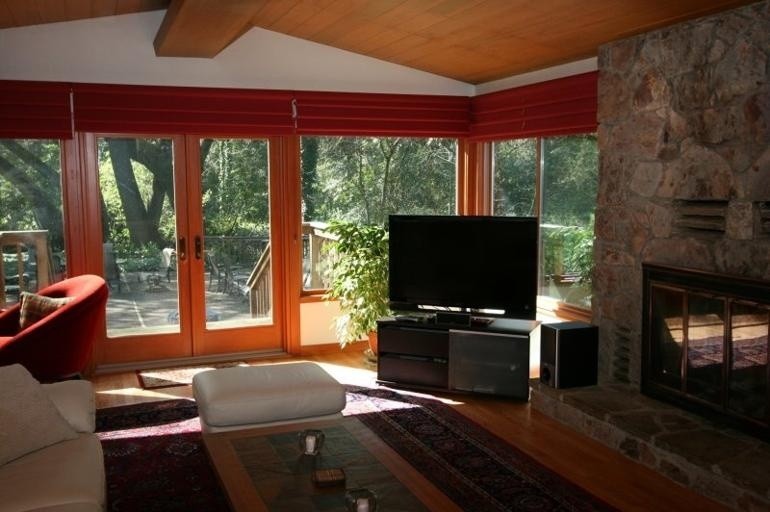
[298,429,324,457]
[345,489,376,512]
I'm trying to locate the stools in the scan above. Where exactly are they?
[193,363,346,434]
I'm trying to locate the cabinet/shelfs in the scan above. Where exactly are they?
[376,320,542,402]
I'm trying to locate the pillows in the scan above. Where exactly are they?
[0,364,79,465]
[17,291,72,333]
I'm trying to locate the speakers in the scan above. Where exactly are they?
[541,321,598,389]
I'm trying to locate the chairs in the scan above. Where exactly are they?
[0,380,107,512]
[103,243,121,295]
[0,273,108,383]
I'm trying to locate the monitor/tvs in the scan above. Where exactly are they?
[388,214,538,321]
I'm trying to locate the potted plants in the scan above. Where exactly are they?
[315,219,407,363]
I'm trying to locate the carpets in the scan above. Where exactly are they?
[135,360,248,389]
[96,384,622,512]
